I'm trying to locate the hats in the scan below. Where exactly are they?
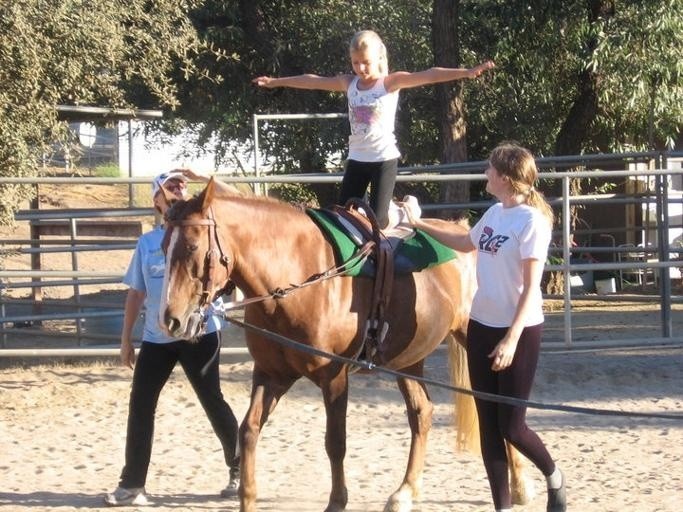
[151,171,188,201]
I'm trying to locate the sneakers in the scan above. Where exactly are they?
[221,467,242,499]
[104,485,150,507]
[546,469,568,512]
[402,194,422,221]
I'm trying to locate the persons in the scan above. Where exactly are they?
[394,144,566,511]
[252,30,495,232]
[105,168,243,506]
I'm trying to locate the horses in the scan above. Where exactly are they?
[157,175,527,512]
